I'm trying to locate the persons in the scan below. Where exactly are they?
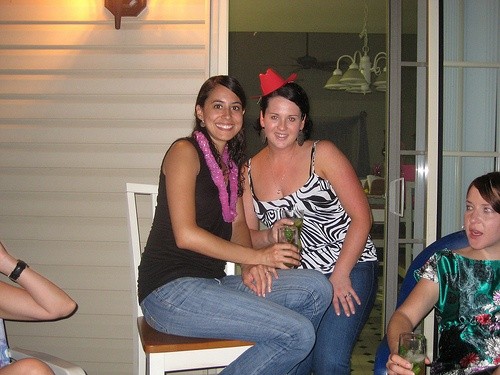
[239,68,379,375]
[137,76,334,375]
[385,171,500,375]
[0,242,77,375]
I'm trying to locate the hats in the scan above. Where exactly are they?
[250,68,297,101]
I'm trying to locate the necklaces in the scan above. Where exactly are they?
[273,173,286,195]
[194,130,239,223]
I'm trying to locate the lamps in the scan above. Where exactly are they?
[104,0,148,29]
[323,0,388,96]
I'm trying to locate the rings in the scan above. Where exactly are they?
[345,293,351,299]
[265,271,270,275]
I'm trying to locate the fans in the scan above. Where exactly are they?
[264,35,349,74]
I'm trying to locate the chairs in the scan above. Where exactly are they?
[126,182,256,375]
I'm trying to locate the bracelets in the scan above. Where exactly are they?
[8,259,28,283]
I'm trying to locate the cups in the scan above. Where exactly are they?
[279,226,299,270]
[397,332,426,375]
[281,209,304,237]
